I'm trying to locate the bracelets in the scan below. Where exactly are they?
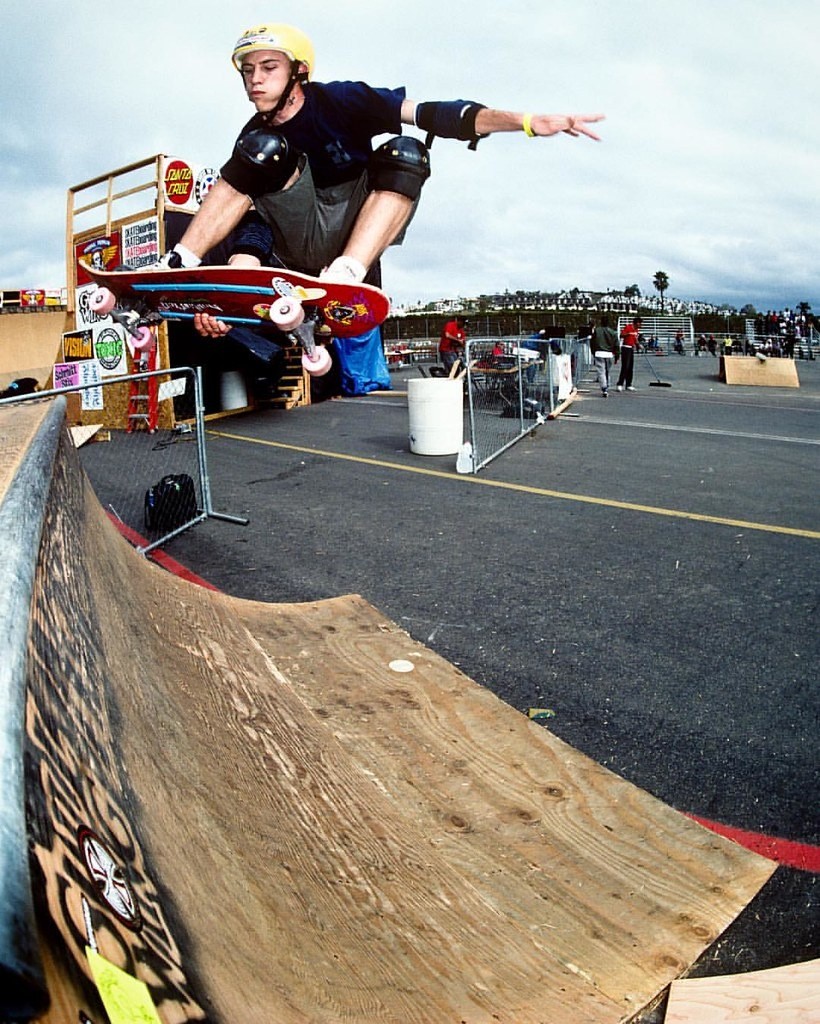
[522,112,538,138]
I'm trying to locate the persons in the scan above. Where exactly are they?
[636,308,814,360]
[113,24,608,338]
[587,315,644,398]
[492,340,519,391]
[438,315,479,395]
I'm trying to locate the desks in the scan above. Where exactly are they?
[468,359,546,407]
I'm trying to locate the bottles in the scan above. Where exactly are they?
[456,441,474,474]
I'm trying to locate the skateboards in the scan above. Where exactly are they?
[78,247,391,378]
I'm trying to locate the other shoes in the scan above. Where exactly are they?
[602,392,608,397]
[626,386,635,391]
[616,385,623,391]
[116,250,187,272]
[317,266,362,284]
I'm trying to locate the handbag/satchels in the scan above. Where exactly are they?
[145,473,197,533]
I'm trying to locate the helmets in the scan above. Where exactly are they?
[232,24,314,83]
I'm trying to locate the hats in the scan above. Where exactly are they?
[460,316,468,325]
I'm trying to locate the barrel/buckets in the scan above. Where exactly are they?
[221,371,248,410]
[407,377,464,456]
